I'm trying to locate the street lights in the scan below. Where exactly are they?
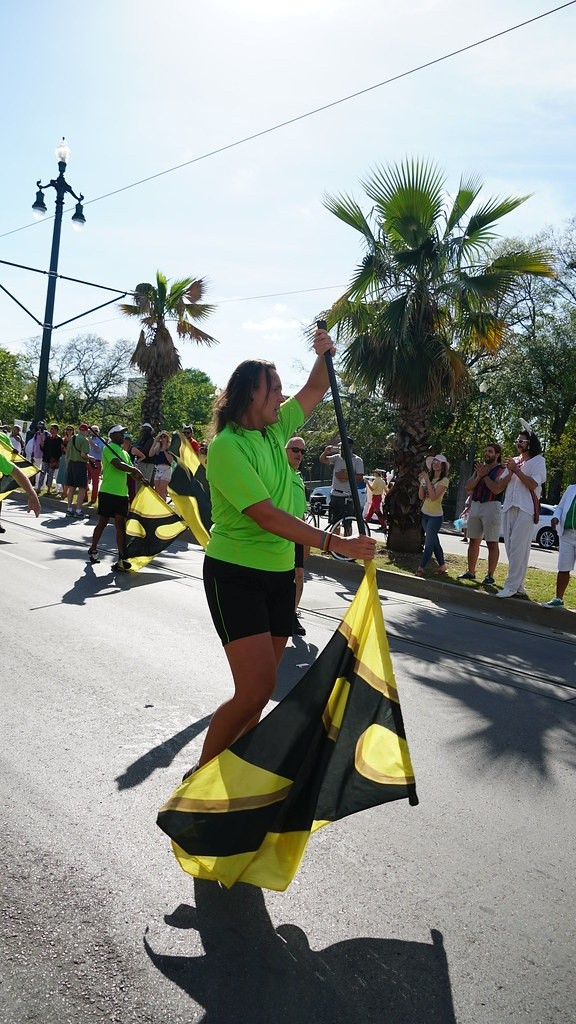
[79,391,88,414]
[467,378,489,474]
[22,395,28,421]
[34,134,86,432]
[59,392,64,421]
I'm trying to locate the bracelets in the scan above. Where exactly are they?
[135,462,138,464]
[515,469,521,474]
[482,475,488,478]
[320,531,332,551]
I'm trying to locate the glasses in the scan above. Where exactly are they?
[432,461,443,465]
[285,447,306,455]
[516,439,529,443]
[67,428,73,431]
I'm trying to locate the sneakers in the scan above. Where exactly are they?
[457,570,476,581]
[482,575,496,585]
[544,598,565,608]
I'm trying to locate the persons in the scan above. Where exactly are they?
[0,433,19,533]
[124,423,209,501]
[496,430,546,597]
[364,469,389,530]
[542,485,576,607]
[457,443,507,585]
[0,455,41,518]
[459,496,470,542]
[319,437,364,554]
[0,421,104,518]
[182,328,377,783]
[414,455,450,576]
[88,425,150,563]
[284,436,306,636]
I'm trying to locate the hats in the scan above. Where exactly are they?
[372,468,382,476]
[79,424,88,431]
[425,455,450,471]
[108,425,128,437]
[488,443,502,464]
[157,431,171,439]
[143,423,154,431]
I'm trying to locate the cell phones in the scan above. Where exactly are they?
[330,446,339,451]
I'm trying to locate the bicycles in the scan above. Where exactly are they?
[304,488,371,560]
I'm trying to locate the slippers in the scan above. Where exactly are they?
[433,569,449,575]
[416,570,423,576]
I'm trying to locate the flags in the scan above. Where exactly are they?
[0,440,40,502]
[167,432,213,550]
[156,557,416,891]
[110,483,187,573]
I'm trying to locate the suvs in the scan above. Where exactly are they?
[309,475,386,516]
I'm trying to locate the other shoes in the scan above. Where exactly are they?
[115,558,130,568]
[88,548,100,563]
[377,527,387,532]
[66,511,74,518]
[496,588,526,598]
[74,510,90,518]
[295,617,306,635]
[461,538,467,542]
[86,499,96,505]
[60,494,67,500]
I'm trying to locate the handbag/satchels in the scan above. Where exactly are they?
[533,497,541,525]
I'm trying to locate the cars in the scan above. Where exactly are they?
[499,503,559,550]
